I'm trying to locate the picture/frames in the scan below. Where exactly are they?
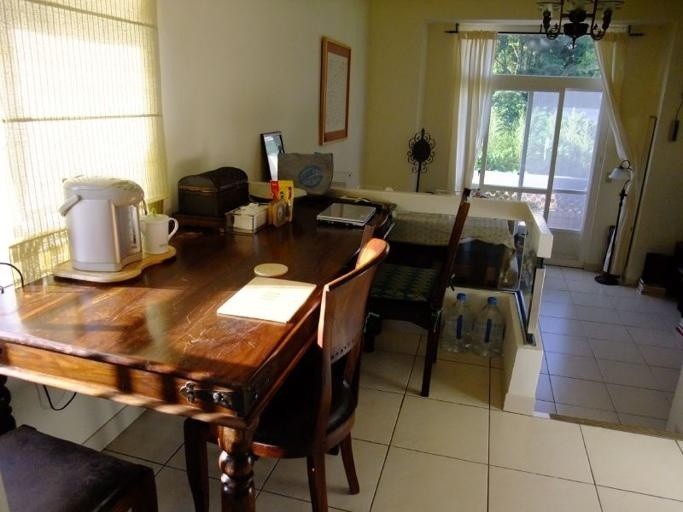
[261,131,285,180]
[319,36,351,146]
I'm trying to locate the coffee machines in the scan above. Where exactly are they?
[60,174,144,273]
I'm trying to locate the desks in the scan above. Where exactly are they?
[328,190,555,415]
[0,194,399,512]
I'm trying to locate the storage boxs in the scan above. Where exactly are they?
[177,167,249,217]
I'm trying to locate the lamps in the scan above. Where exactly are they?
[537,0,613,49]
[594,160,634,286]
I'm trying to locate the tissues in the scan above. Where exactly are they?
[231,202,268,234]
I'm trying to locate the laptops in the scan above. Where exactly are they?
[316,202,377,227]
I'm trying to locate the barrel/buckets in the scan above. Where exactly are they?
[441,292,473,353]
[473,295,504,358]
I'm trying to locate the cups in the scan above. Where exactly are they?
[140,208,178,255]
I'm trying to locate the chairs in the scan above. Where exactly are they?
[365,188,471,397]
[0,424,158,511]
[184,239,391,512]
